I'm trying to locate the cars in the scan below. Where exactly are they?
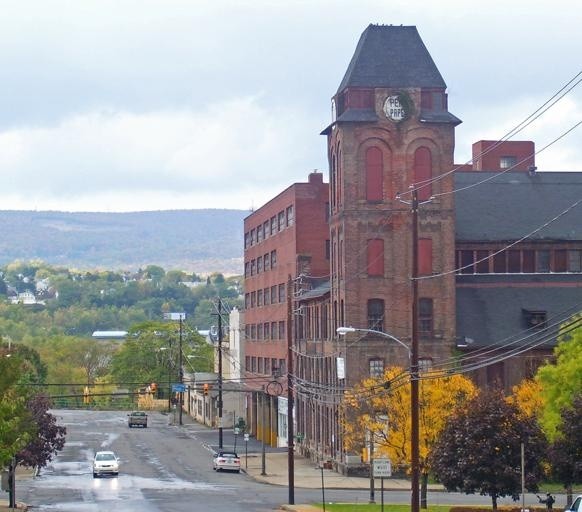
[127,411,148,427]
[213,451,241,472]
[93,450,121,477]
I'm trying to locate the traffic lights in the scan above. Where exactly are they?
[151,383,158,393]
[203,383,209,396]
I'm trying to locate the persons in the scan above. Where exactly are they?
[170,396,176,410]
